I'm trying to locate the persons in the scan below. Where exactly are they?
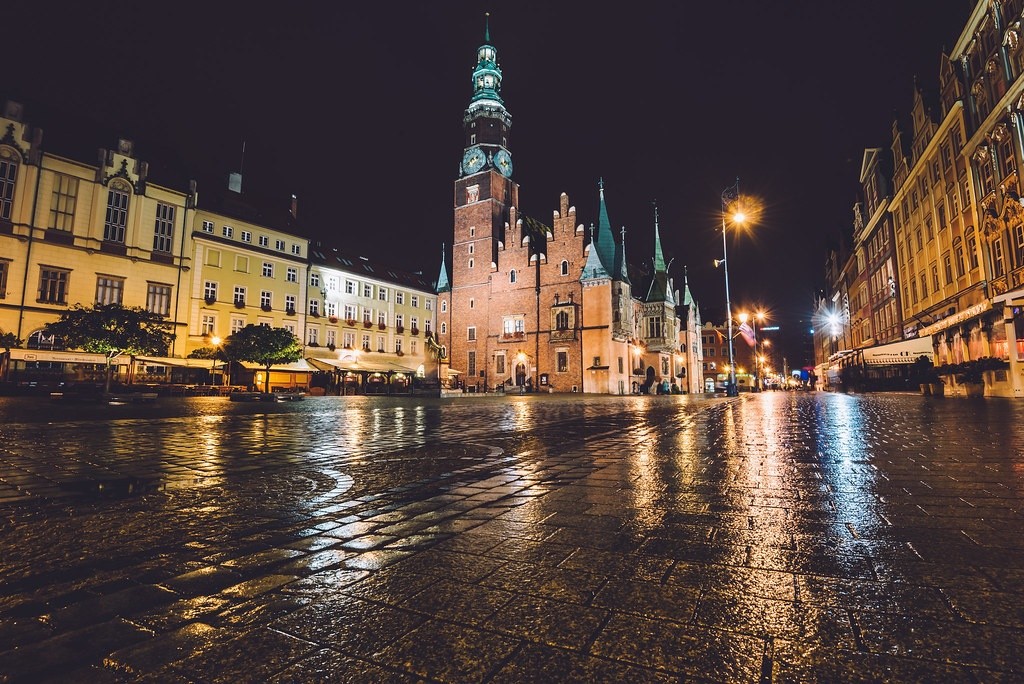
[801,368,815,389]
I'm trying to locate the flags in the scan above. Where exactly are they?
[738,323,757,348]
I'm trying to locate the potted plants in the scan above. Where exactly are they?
[949,356,1011,398]
[909,355,947,397]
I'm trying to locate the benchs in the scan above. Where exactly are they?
[147,384,248,397]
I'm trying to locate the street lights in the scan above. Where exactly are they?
[519,353,526,395]
[719,176,748,396]
[211,336,219,385]
